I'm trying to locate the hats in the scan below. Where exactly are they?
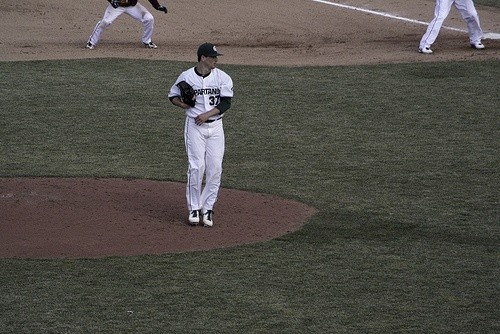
[198,42,224,56]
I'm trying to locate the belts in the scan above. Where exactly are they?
[204,117,222,123]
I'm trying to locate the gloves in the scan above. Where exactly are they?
[159,6,167,13]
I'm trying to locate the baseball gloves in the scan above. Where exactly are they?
[175,80,196,107]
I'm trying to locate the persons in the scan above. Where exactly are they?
[86,0,167,51]
[168,42,233,227]
[418,0,485,53]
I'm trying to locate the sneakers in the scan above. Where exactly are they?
[147,42,157,48]
[189,210,199,225]
[200,210,214,227]
[469,42,484,48]
[419,46,433,54]
[87,43,94,50]
